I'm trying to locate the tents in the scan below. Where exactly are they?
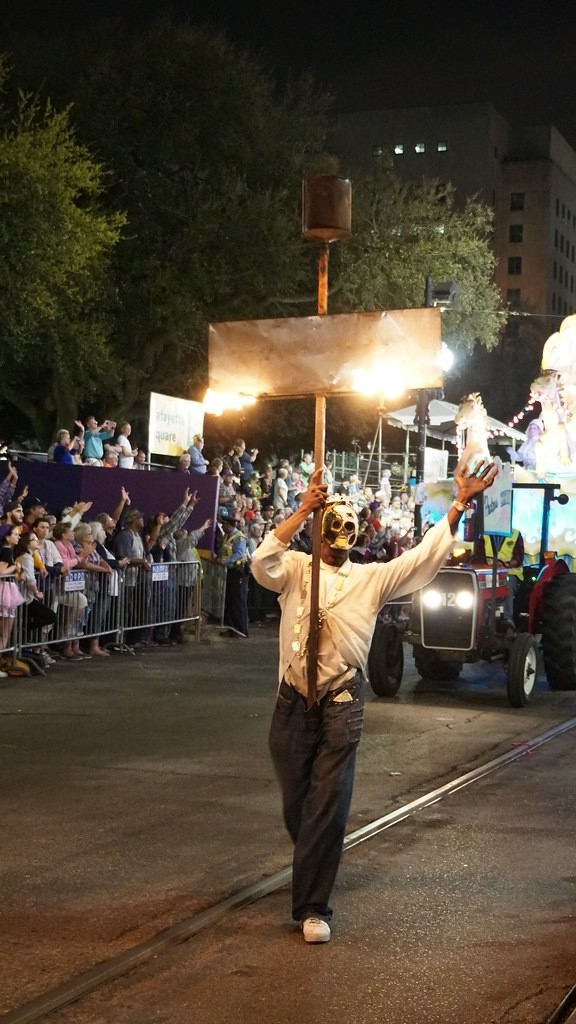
[362,399,528,490]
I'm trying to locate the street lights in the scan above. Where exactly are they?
[413,342,450,547]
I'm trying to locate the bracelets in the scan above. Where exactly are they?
[452,500,469,512]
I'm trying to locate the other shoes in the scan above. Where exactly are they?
[225,632,249,638]
[220,629,236,635]
[0,625,191,679]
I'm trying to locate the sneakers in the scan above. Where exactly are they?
[305,914,331,942]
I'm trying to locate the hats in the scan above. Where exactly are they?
[0,501,23,523]
[530,419,543,433]
[219,514,239,526]
[254,515,268,524]
[222,469,235,478]
[121,509,144,525]
[24,497,46,506]
[263,504,276,511]
[244,511,256,520]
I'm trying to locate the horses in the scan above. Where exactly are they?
[454,391,490,463]
[530,367,574,472]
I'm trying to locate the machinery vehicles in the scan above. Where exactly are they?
[368,482,576,707]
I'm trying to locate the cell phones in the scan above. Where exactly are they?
[266,493,271,496]
[106,421,114,424]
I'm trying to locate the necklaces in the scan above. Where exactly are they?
[292,558,345,659]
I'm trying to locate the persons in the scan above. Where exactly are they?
[506,419,543,470]
[250,460,499,942]
[478,528,524,631]
[0,433,417,677]
[53,414,146,470]
